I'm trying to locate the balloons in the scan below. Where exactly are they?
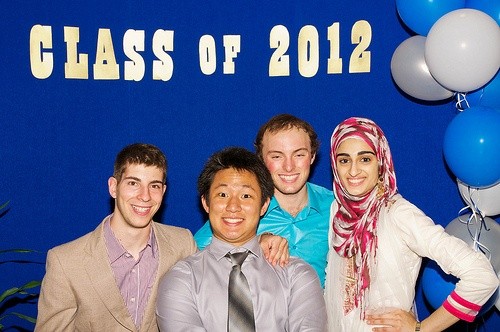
[456,179,500,217]
[456,68,499,105]
[444,211,499,272]
[423,261,497,318]
[477,310,500,332]
[442,106,500,187]
[425,8,500,94]
[462,0,500,28]
[390,37,456,100]
[396,1,465,36]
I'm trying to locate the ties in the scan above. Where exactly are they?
[224,250,257,332]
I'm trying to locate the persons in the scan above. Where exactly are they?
[194,114,334,293]
[323,117,499,332]
[34,144,290,332]
[156,146,329,332]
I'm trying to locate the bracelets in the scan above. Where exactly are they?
[258,231,274,243]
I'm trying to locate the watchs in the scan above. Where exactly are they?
[416,321,421,332]
